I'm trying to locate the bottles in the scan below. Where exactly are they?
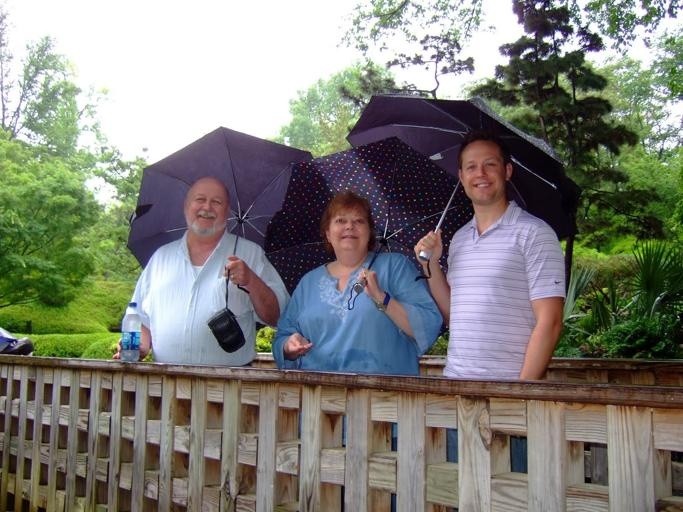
[119,301,140,362]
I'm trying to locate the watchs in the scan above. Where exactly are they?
[375,290,391,313]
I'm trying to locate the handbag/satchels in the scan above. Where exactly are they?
[212,310,246,352]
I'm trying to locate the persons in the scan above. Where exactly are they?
[271,188,443,512]
[412,135,565,473]
[110,174,291,371]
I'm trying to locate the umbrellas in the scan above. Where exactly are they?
[340,91,580,262]
[127,125,316,271]
[264,132,477,299]
